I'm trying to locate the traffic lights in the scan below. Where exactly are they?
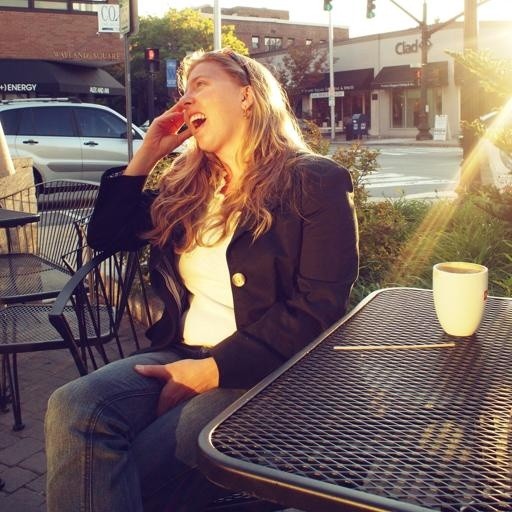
[324,0,333,10]
[144,47,160,75]
[368,0,376,19]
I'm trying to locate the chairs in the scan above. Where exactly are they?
[0,180,99,414]
[48,247,288,512]
[0,245,139,431]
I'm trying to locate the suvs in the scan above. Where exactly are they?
[0,98,188,198]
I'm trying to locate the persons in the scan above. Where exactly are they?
[45,46,361,512]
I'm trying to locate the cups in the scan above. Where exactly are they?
[431,261,490,338]
